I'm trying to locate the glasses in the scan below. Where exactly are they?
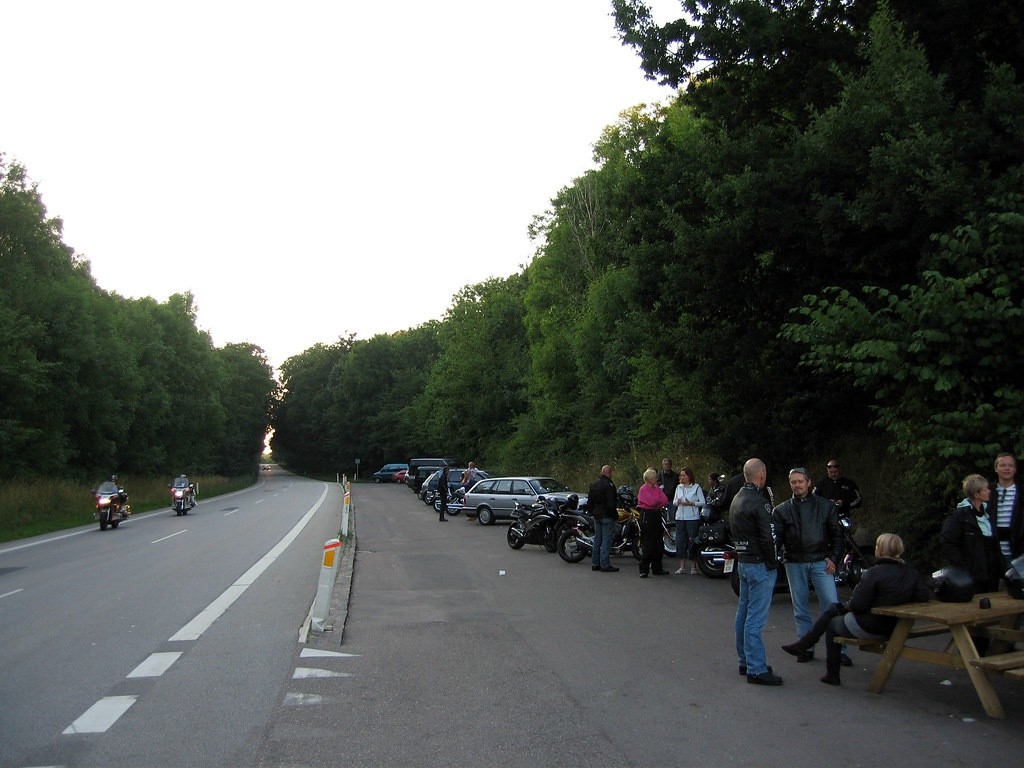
[826,464,838,469]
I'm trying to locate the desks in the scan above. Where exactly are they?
[871,593,1024,720]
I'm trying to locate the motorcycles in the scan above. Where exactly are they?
[424,488,463,516]
[507,494,579,550]
[557,485,678,563]
[688,497,878,591]
[91,481,128,530]
[168,477,196,515]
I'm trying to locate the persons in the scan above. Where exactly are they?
[461,462,478,521]
[813,460,861,516]
[638,467,670,578]
[171,475,193,503]
[657,458,679,520]
[772,468,852,667]
[700,472,725,525]
[987,452,1024,570]
[439,467,450,522]
[781,533,923,685]
[729,458,784,686]
[98,475,128,513]
[941,474,1009,659]
[588,465,619,572]
[673,467,705,575]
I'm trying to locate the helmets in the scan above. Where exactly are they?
[568,494,579,510]
[108,475,119,481]
[181,474,187,478]
[1004,555,1024,600]
[932,565,974,604]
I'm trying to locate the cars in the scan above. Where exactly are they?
[392,470,408,483]
[422,469,492,500]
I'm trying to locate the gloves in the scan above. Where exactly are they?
[830,499,844,507]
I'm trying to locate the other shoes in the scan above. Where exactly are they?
[439,518,448,522]
[674,568,686,575]
[840,654,853,667]
[690,568,698,575]
[819,675,841,685]
[781,644,808,657]
[601,565,620,572]
[591,565,602,571]
[738,663,773,676]
[746,673,782,686]
[639,573,649,578]
[652,569,669,575]
[796,650,814,663]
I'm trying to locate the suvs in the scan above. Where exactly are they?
[462,477,590,525]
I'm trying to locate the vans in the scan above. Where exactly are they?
[408,458,464,492]
[415,466,460,495]
[373,463,408,483]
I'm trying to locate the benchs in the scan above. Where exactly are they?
[833,624,1024,680]
